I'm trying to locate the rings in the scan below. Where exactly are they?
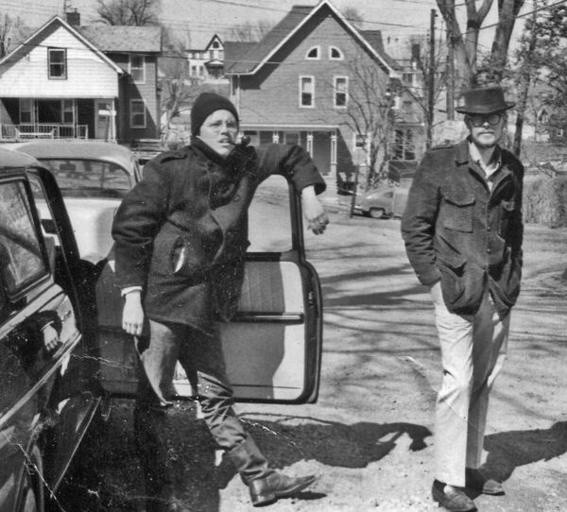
[319,221,323,224]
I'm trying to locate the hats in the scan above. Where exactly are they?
[454,85,517,116]
[188,91,240,138]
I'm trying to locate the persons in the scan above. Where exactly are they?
[108,89,332,512]
[7,310,62,497]
[399,86,524,512]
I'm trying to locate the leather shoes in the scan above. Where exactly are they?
[468,470,506,496]
[430,479,477,512]
[249,471,322,508]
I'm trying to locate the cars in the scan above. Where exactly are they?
[354,187,395,219]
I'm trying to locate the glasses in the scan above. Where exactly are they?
[466,113,505,128]
